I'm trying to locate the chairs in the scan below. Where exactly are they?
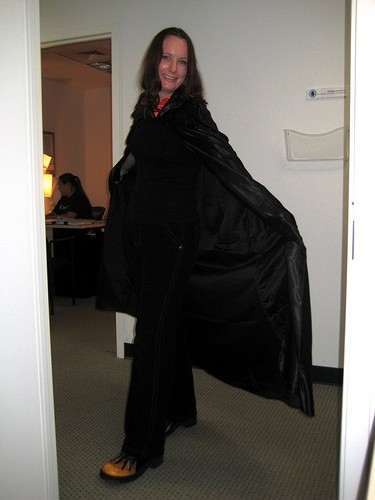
[90,206,105,221]
[46,236,76,316]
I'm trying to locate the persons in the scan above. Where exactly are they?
[91,28,316,482]
[45,173,93,219]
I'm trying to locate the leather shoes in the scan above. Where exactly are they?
[100,451,162,484]
[165,413,198,435]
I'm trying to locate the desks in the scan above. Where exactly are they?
[45,217,107,316]
